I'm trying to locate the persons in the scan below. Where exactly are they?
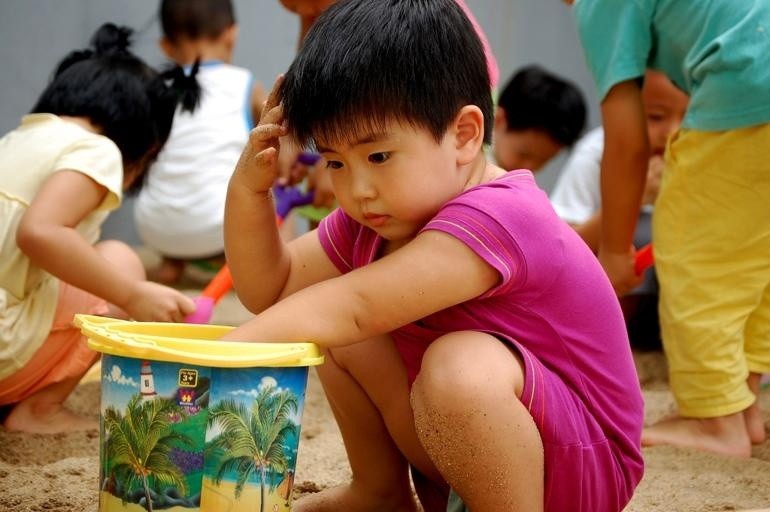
[222,1,647,511]
[559,0,769,464]
[276,0,498,209]
[0,19,202,440]
[551,70,691,351]
[488,64,588,198]
[135,1,269,283]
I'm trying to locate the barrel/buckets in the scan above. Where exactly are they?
[71,313,325,512]
